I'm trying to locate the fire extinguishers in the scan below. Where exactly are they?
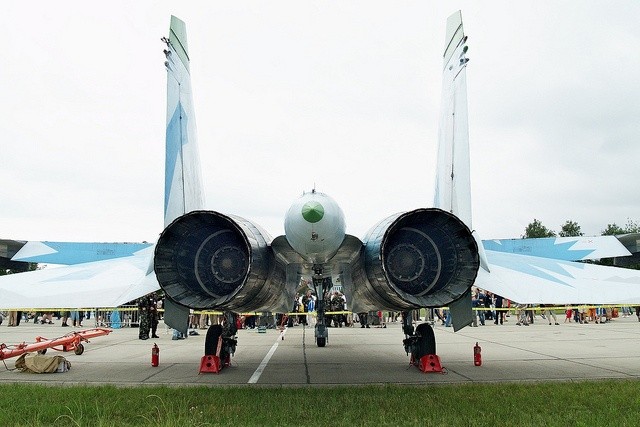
[474,341,481,366]
[152,342,159,367]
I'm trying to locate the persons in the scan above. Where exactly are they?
[514,302,537,326]
[147,290,162,338]
[425,308,452,327]
[157,295,165,320]
[0,299,138,327]
[324,290,382,327]
[298,291,318,325]
[388,311,422,325]
[139,292,151,340]
[470,288,510,327]
[189,299,297,327]
[540,304,559,325]
[564,304,640,324]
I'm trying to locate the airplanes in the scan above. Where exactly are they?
[0,7,640,370]
